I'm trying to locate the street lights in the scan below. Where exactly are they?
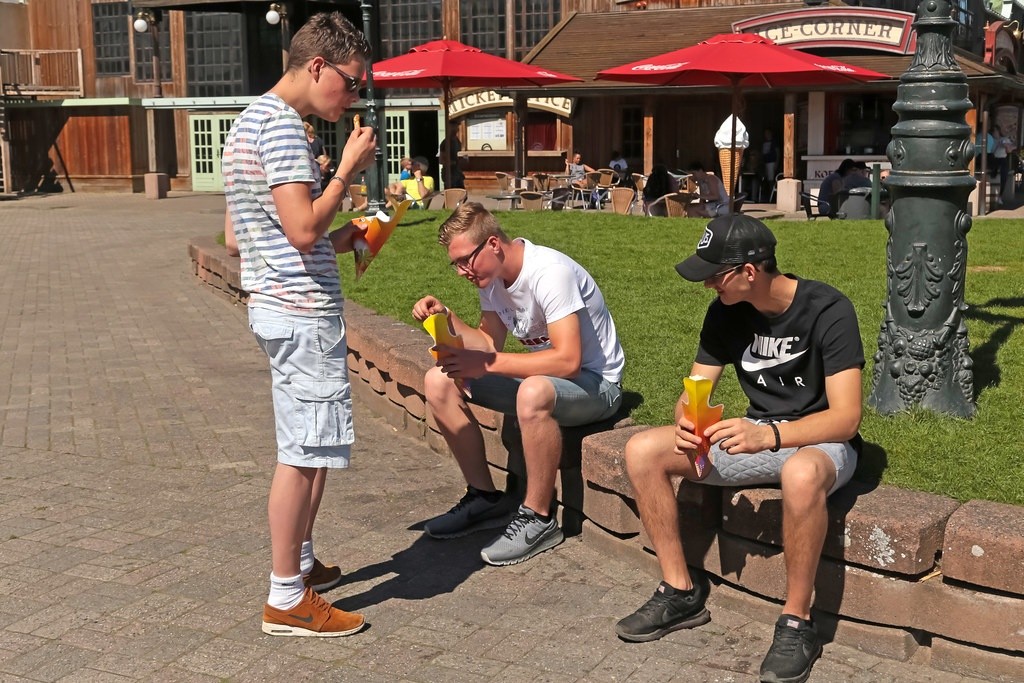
[132,12,165,97]
[265,2,291,79]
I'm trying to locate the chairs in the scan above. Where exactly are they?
[799,191,833,221]
[494,169,784,218]
[348,184,468,209]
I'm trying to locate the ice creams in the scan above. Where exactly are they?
[713,113,750,198]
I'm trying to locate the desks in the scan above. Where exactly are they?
[485,191,521,210]
[552,175,576,188]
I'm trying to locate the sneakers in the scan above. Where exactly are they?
[480,504,563,566]
[424,486,512,539]
[759,612,821,683]
[302,556,341,591]
[261,586,366,637]
[614,581,710,642]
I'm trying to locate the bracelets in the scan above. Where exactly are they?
[766,423,780,452]
[330,176,348,189]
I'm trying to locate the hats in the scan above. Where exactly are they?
[675,213,776,282]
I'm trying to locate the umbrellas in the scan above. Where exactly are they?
[362,35,585,187]
[595,30,895,214]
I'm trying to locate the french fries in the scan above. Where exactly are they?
[353,114,360,129]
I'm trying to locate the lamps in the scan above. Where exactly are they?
[266,3,287,25]
[1003,20,1021,39]
[134,11,155,32]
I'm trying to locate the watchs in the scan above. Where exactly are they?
[416,178,423,181]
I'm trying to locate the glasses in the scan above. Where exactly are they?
[308,60,361,92]
[710,261,760,283]
[450,234,498,271]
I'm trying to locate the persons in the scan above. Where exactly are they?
[609,151,627,171]
[686,162,729,217]
[383,157,434,209]
[761,129,779,162]
[990,126,1013,205]
[817,159,872,216]
[412,202,625,566]
[439,118,473,190]
[564,153,604,205]
[302,121,332,193]
[615,214,867,683]
[222,13,372,637]
